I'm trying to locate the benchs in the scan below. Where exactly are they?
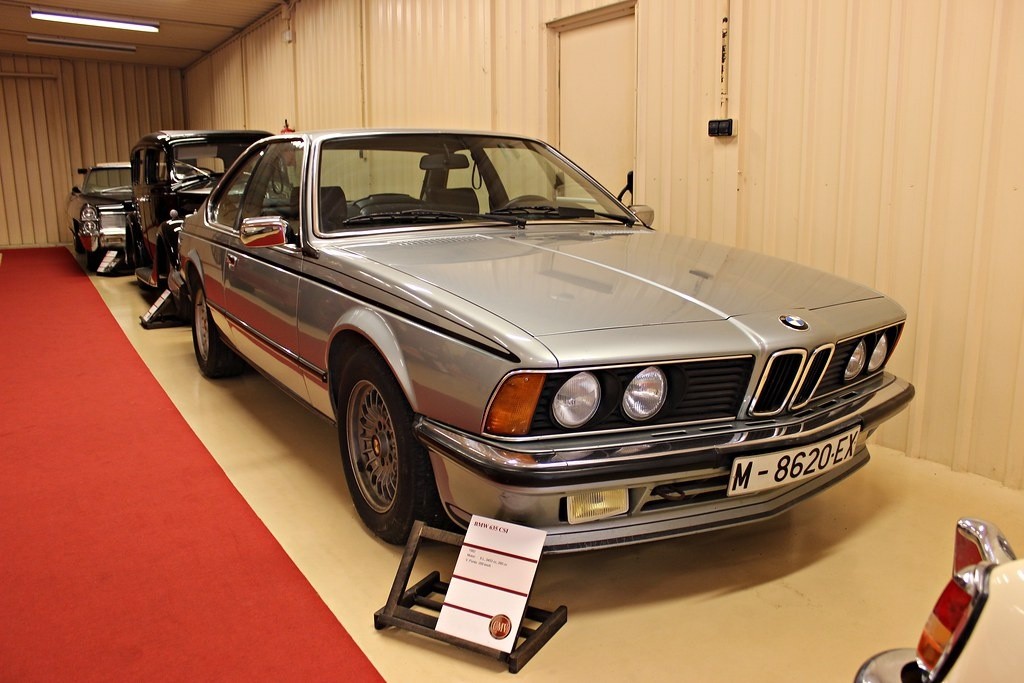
[262,193,422,220]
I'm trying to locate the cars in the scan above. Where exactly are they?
[67,161,133,271]
[854,519,1023,683]
[179,128,917,546]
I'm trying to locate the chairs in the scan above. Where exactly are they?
[425,188,481,214]
[290,186,347,231]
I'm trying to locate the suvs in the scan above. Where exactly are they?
[121,128,297,325]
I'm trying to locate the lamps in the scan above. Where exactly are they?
[27,34,136,54]
[28,5,160,33]
